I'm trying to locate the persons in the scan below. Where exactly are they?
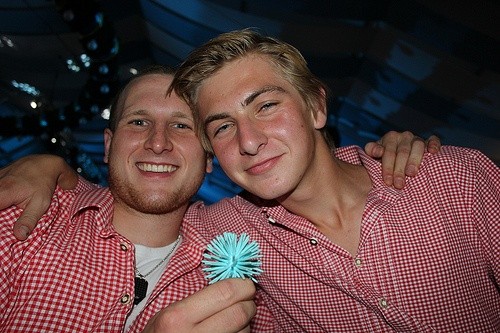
[1,63,442,333]
[0,26,500,333]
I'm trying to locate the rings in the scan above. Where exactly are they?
[410,136,428,148]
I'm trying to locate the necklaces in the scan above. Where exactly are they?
[134,234,182,306]
